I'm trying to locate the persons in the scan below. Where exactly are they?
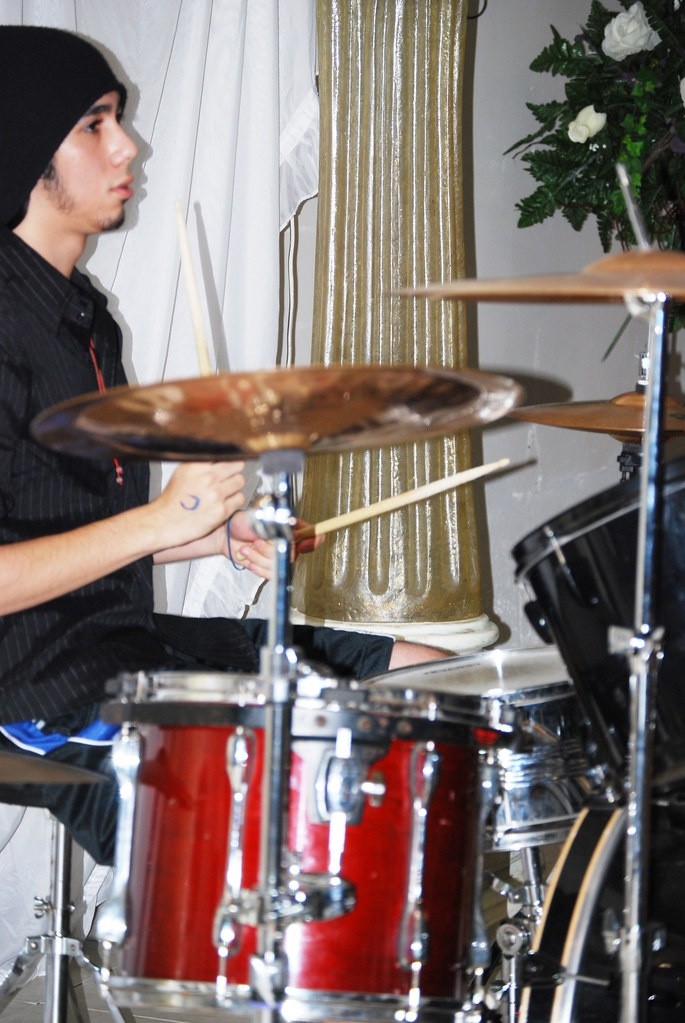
[0,0,463,867]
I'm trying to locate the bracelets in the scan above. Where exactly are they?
[226,515,247,570]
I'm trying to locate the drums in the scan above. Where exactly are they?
[99,467,685,1023]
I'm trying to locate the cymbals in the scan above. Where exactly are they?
[29,251,685,463]
[0,749,112,784]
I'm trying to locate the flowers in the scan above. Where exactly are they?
[502,1,685,335]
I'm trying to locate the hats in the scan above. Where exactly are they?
[0,26,125,228]
